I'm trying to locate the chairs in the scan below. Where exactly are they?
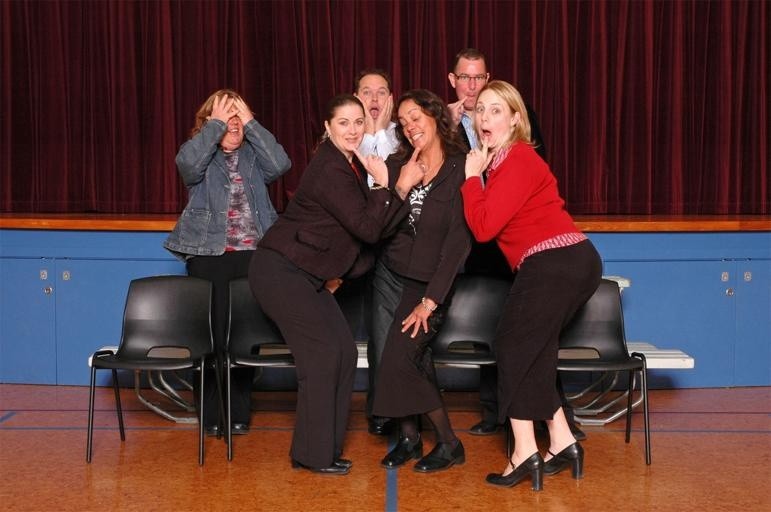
[430,273,512,463]
[330,271,386,369]
[224,276,297,461]
[507,278,652,466]
[85,276,223,466]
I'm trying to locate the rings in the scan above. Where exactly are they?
[470,151,476,155]
[419,163,426,168]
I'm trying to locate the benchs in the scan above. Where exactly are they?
[87,340,695,427]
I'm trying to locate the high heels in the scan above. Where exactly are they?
[486,449,545,491]
[415,438,466,472]
[543,441,584,479]
[381,433,424,469]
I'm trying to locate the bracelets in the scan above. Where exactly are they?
[421,296,438,312]
[370,183,390,190]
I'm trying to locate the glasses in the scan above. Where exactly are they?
[452,72,488,83]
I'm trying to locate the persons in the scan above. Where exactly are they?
[162,89,292,437]
[372,88,476,473]
[460,80,603,491]
[353,67,415,436]
[247,94,392,475]
[445,47,586,442]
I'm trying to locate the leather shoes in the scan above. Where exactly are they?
[231,423,251,435]
[203,424,223,437]
[370,417,399,436]
[470,411,509,435]
[291,456,349,474]
[535,420,587,440]
[332,457,352,468]
